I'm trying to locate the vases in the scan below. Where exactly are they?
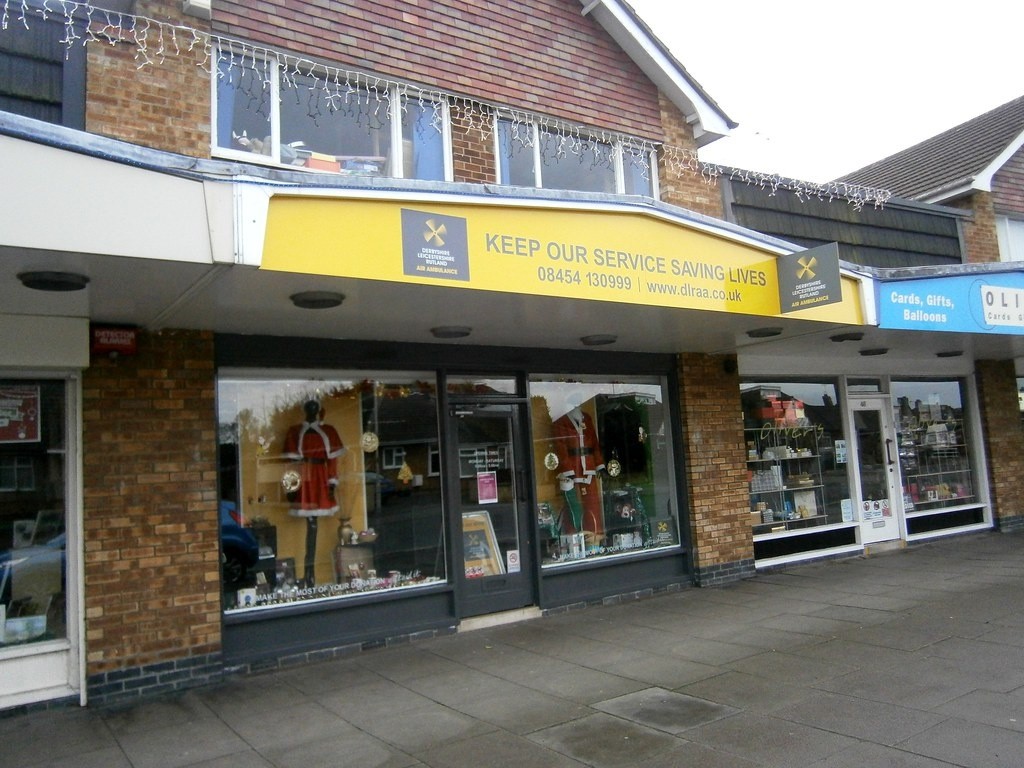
[337,516,354,545]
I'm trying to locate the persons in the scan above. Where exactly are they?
[281,393,345,517]
[551,384,610,556]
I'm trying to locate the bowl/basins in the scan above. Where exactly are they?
[359,534,378,542]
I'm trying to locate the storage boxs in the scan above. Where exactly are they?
[560,533,586,561]
[747,437,818,525]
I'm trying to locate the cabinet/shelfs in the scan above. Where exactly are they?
[746,425,828,534]
[898,418,976,512]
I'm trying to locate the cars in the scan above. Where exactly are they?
[0,498,263,624]
